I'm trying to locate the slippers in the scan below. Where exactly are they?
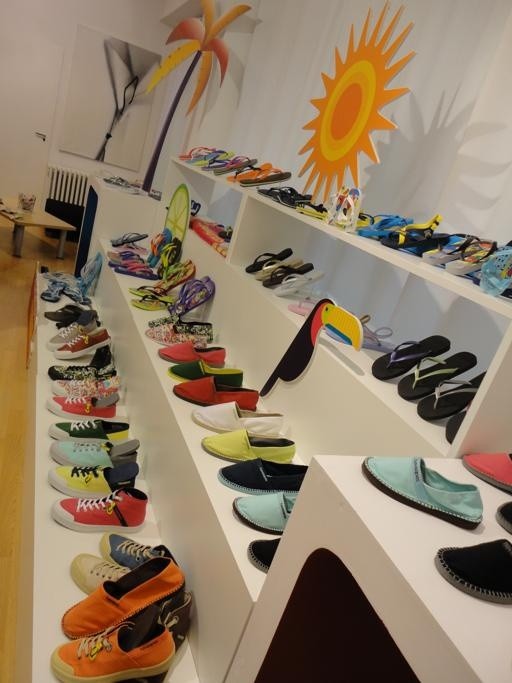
[110,233,150,253]
[178,146,291,185]
[150,258,196,294]
[263,262,313,288]
[103,174,162,200]
[275,269,325,297]
[245,247,293,273]
[288,298,395,353]
[371,334,451,380]
[40,250,101,327]
[148,228,181,278]
[257,186,311,209]
[255,256,302,282]
[191,401,281,433]
[107,251,157,280]
[417,371,489,443]
[295,184,512,295]
[169,275,215,315]
[128,285,177,311]
[397,352,478,399]
[165,200,201,216]
[208,221,233,242]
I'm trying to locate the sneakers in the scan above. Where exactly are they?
[50,487,148,533]
[53,376,119,396]
[99,531,178,571]
[50,593,193,683]
[46,392,119,418]
[72,553,131,595]
[48,361,116,381]
[50,420,131,440]
[48,463,139,498]
[49,439,141,471]
[54,328,111,359]
[47,318,99,351]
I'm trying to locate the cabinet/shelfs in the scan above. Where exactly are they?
[23,148,512,683]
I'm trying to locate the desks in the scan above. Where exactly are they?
[0,193,77,260]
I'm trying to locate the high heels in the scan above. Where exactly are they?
[494,502,512,536]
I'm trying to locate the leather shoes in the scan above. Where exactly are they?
[434,539,512,604]
[202,429,295,465]
[362,452,484,529]
[159,343,224,366]
[464,450,512,492]
[173,376,259,410]
[167,357,242,389]
[148,315,214,342]
[60,556,184,637]
[148,323,206,346]
[233,492,294,534]
[217,457,308,492]
[246,539,280,571]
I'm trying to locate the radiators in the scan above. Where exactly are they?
[46,163,89,208]
